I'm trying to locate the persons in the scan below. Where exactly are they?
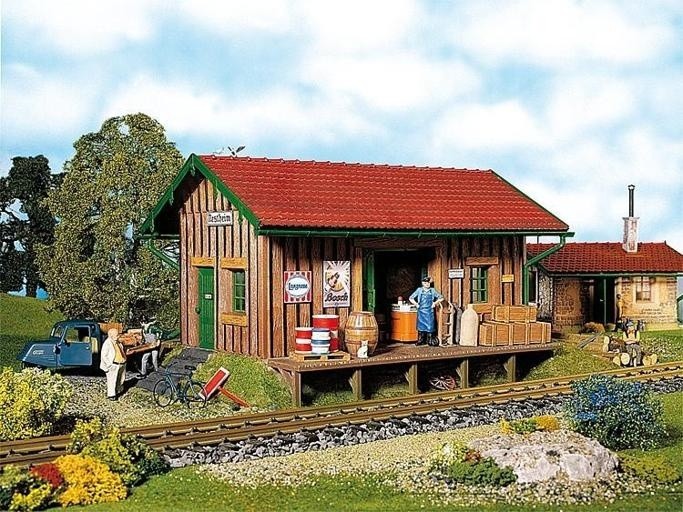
[612,293,629,338]
[99,327,128,401]
[135,314,164,377]
[623,320,644,368]
[407,276,445,345]
